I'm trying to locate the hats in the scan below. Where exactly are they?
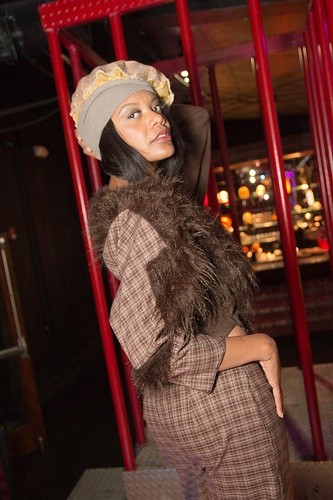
[69,60,174,162]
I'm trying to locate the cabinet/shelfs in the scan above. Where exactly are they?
[211,148,329,263]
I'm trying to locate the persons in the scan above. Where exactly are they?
[68,60,291,500]
